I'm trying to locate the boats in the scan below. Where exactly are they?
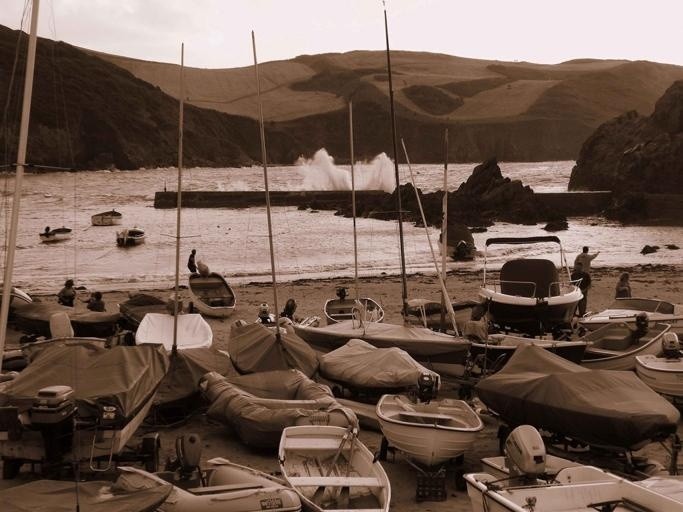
[92,211,123,226]
[229,319,318,382]
[0,463,173,512]
[400,299,480,330]
[227,327,319,381]
[318,338,441,393]
[437,240,477,259]
[91,209,123,225]
[377,370,484,468]
[138,346,233,425]
[9,297,124,334]
[188,271,237,317]
[116,226,146,247]
[188,262,236,319]
[0,287,34,308]
[15,301,121,339]
[116,229,145,246]
[465,320,586,365]
[376,392,482,466]
[317,339,441,402]
[151,347,232,410]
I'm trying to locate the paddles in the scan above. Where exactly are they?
[336,422,358,509]
[311,422,351,506]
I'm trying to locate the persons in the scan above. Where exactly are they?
[571,262,591,318]
[574,247,600,274]
[58,280,77,307]
[614,273,632,299]
[187,249,198,272]
[87,292,106,312]
